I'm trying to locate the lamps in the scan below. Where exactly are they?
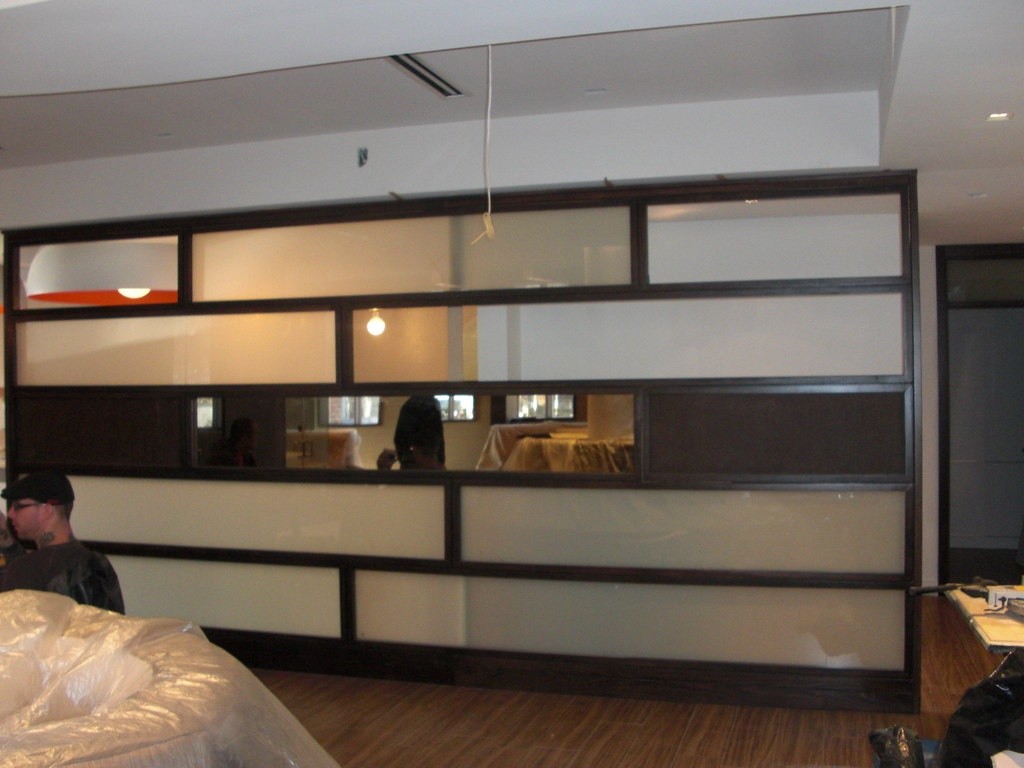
[366,309,386,335]
[27,241,177,305]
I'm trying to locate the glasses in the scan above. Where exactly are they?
[13,499,40,512]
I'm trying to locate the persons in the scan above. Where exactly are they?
[198,418,258,467]
[394,392,445,472]
[0,468,125,614]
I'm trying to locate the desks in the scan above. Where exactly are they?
[944,583,1024,679]
[287,428,362,469]
[0,590,341,768]
[501,435,634,473]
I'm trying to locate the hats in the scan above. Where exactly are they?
[1,470,74,506]
